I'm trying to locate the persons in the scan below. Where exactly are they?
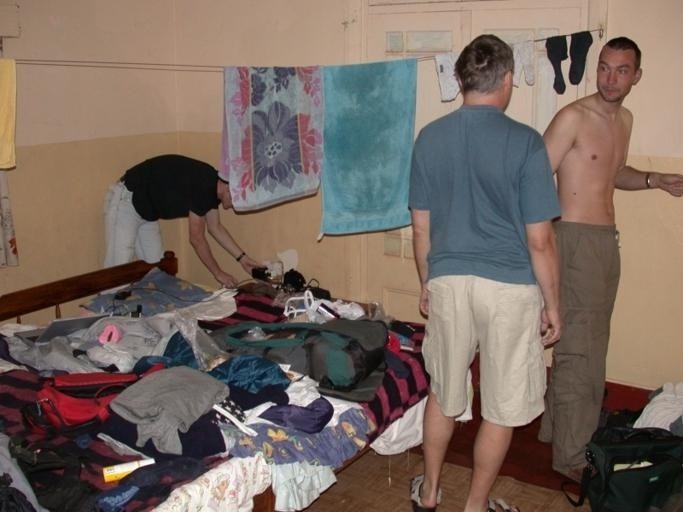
[407,34,564,512]
[102,153,267,293]
[537,38,682,483]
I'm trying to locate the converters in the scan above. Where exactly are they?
[114,291,130,300]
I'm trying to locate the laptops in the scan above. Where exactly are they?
[14,312,109,346]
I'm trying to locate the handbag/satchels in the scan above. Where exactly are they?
[586,425,683,512]
[304,318,389,392]
[487,497,520,512]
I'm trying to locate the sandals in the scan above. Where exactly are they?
[410,473,442,512]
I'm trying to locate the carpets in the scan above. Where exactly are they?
[404,352,650,496]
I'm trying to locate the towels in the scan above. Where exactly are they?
[216,57,417,242]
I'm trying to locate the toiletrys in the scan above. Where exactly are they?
[103,458,156,483]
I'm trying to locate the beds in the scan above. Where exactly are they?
[149,279,430,512]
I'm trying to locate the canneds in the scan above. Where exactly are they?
[316,303,340,320]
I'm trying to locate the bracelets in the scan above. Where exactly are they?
[236,252,246,262]
[646,172,651,190]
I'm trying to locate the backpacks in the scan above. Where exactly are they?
[0,363,201,512]
